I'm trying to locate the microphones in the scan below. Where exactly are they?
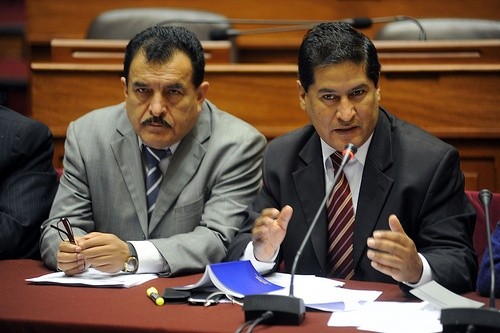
[241,142,358,323]
[439,188,500,333]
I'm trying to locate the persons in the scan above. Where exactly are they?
[40,26,267,278]
[221,22,478,298]
[0,105,58,260]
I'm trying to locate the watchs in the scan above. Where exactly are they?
[122,242,138,273]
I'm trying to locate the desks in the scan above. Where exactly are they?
[32,63,500,200]
[0,255,500,333]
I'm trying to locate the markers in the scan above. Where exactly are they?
[147,287,164,305]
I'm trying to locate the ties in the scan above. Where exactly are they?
[142,145,172,227]
[326,151,355,280]
